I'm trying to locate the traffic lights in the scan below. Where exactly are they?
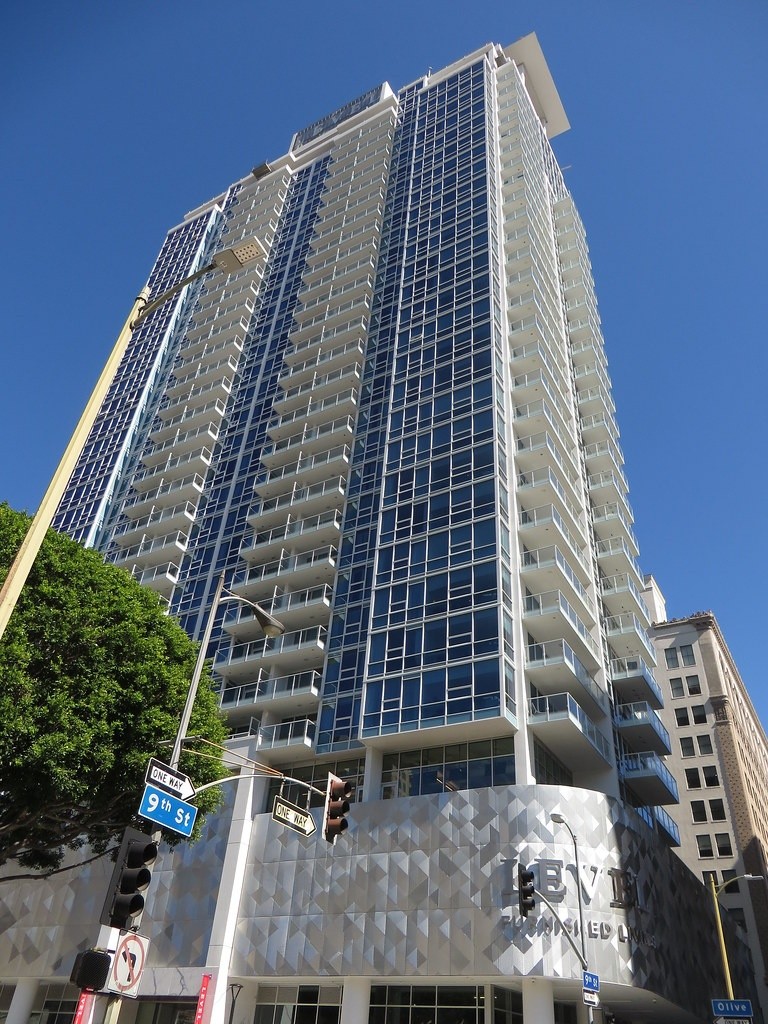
[100,827,160,926]
[321,772,350,847]
[516,863,535,917]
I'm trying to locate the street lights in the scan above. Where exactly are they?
[708,870,764,1024]
[75,566,287,1024]
[548,811,601,1024]
